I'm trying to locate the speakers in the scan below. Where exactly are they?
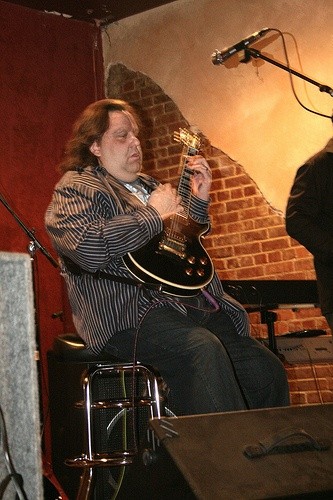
[116,402,333,500]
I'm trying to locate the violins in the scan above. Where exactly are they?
[120,127,214,296]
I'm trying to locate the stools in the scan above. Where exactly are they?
[64,361,170,500]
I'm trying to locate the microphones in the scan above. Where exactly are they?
[211,27,271,65]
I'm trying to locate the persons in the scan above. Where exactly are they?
[44,98,290,413]
[284,138,333,335]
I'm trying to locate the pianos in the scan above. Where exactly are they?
[219,280,325,311]
[256,330,333,366]
